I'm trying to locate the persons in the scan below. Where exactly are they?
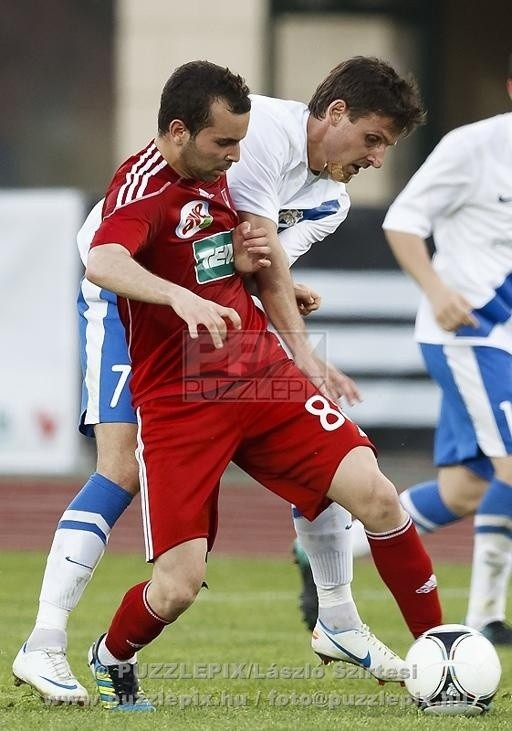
[12,55,429,706]
[291,72,511,661]
[84,60,444,709]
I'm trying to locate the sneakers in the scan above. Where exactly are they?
[87,632,154,713]
[12,642,87,707]
[292,539,318,631]
[309,617,412,684]
[463,619,512,646]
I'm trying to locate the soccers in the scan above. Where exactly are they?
[404,624,502,717]
